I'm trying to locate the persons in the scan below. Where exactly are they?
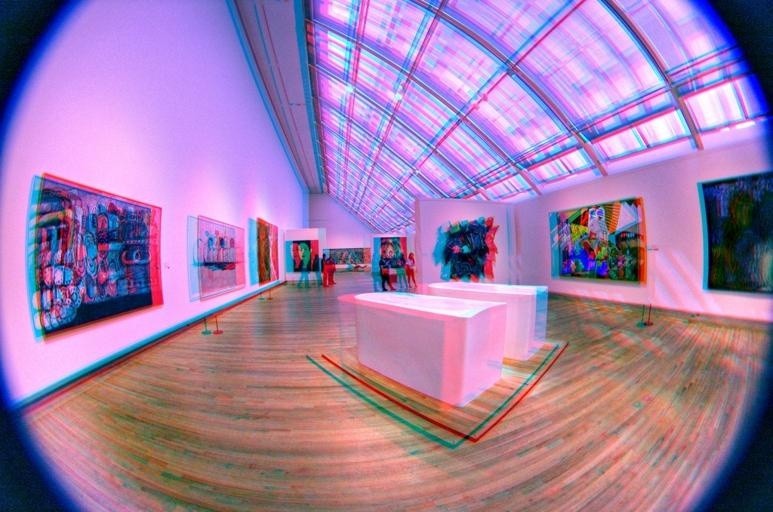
[370,253,417,292]
[295,253,336,287]
